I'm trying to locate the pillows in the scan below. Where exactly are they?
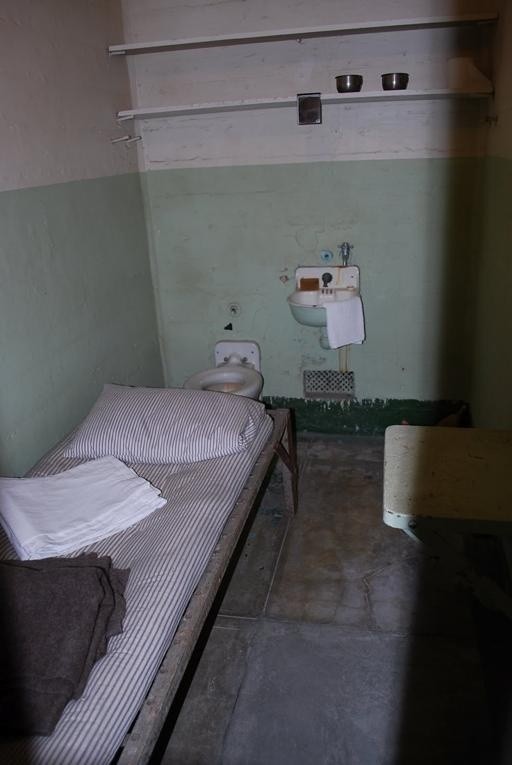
[63,383,265,464]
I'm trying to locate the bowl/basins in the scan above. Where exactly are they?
[381,72,409,90]
[335,75,363,93]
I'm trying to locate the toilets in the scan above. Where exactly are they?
[287,289,360,327]
[182,339,264,403]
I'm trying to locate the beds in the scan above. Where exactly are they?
[1,406,299,765]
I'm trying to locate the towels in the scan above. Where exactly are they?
[321,295,366,349]
[1,454,167,561]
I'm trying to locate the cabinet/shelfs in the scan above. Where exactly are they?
[106,9,499,121]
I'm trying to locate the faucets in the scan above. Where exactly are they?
[337,241,353,265]
[322,273,332,286]
